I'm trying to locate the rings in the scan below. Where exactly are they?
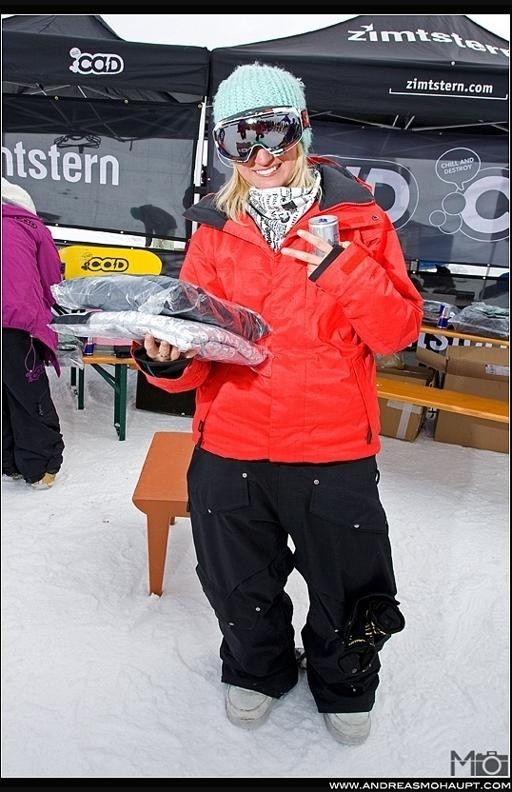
[158,352,169,357]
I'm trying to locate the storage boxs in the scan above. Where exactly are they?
[416,345,509,454]
[376,364,436,443]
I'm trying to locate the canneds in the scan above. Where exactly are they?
[308,215,340,256]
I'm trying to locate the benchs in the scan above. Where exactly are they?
[376,376,509,424]
[132,432,196,597]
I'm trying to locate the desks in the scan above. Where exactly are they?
[419,325,509,348]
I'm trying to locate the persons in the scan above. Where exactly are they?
[131,63,425,747]
[132,205,179,250]
[2,176,62,489]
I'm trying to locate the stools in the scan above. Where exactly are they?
[64,354,136,441]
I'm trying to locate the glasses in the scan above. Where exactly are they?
[212,106,309,164]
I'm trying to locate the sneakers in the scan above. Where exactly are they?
[2,469,55,491]
[226,681,277,728]
[322,711,370,746]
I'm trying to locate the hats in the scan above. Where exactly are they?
[212,65,313,152]
